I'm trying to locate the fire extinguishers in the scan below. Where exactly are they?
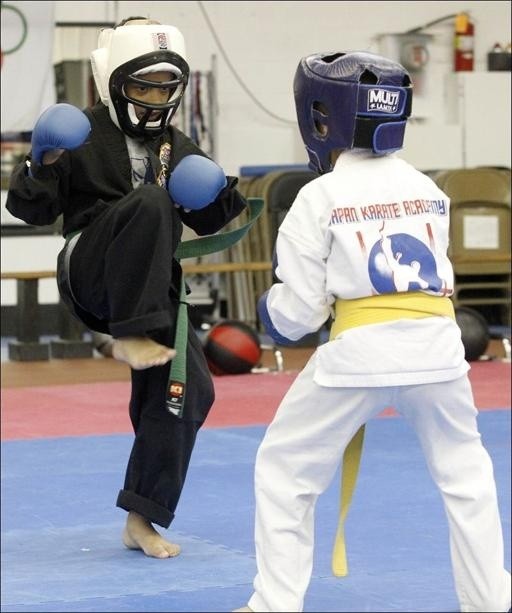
[448,11,477,73]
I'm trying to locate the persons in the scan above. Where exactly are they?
[232,48,511,612]
[6,13,243,560]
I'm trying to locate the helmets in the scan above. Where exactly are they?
[91,17,190,142]
[293,49,413,174]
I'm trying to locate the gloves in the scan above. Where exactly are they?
[256,289,319,347]
[168,154,227,211]
[28,103,92,189]
